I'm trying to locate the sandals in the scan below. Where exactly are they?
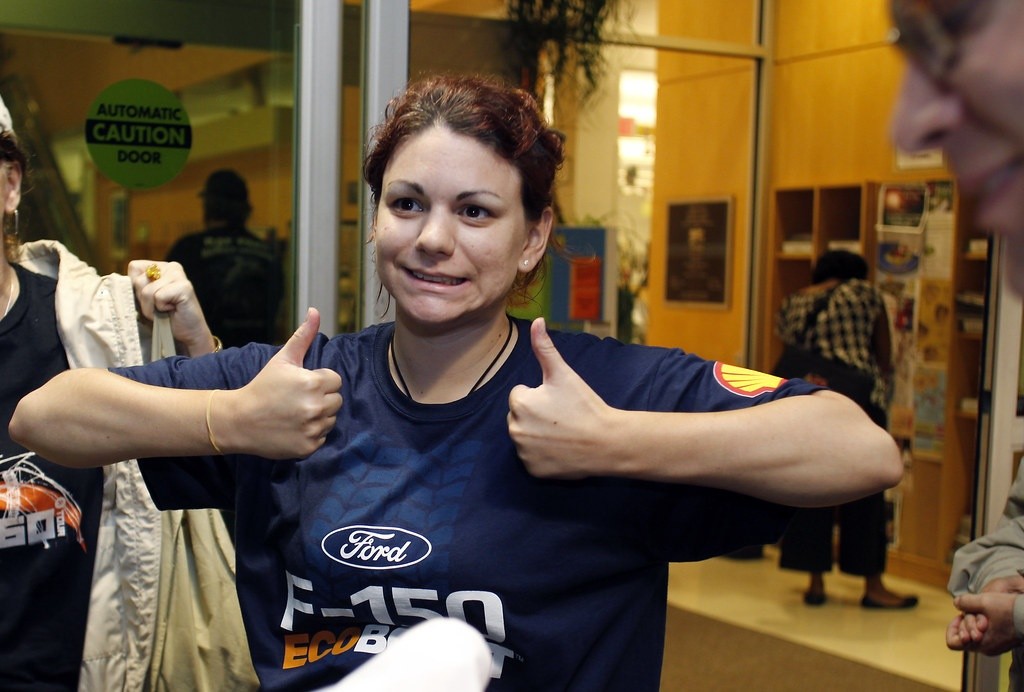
[862,594,917,608]
[802,591,829,606]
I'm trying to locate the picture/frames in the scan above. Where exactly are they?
[662,193,734,312]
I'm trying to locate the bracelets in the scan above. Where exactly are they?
[210,335,222,353]
[207,389,226,455]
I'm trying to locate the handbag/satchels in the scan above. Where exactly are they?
[774,347,873,407]
[146,306,263,692]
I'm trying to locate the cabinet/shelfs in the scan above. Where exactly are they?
[759,180,880,388]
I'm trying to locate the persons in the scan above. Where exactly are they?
[1,98,222,692]
[15,70,904,692]
[776,250,920,609]
[163,168,284,353]
[945,448,1024,691]
[884,1,1024,301]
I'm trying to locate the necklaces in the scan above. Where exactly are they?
[391,315,514,403]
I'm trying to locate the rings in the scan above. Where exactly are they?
[146,265,160,281]
